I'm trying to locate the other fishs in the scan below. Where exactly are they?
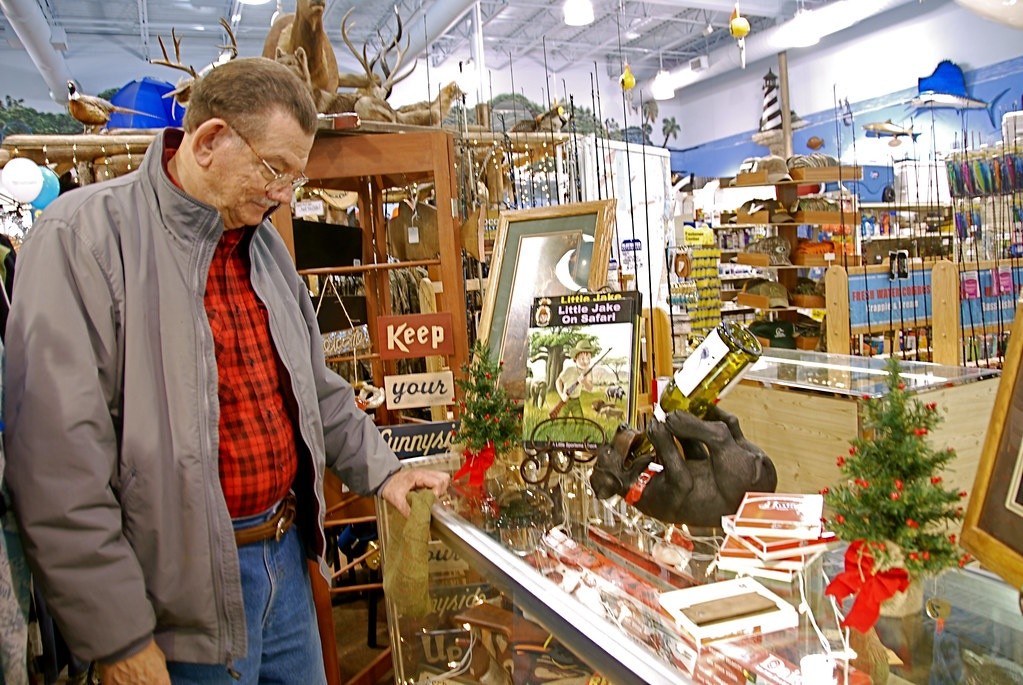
[862,119,914,140]
[904,88,1012,129]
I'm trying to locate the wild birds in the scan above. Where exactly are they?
[396,82,462,126]
[511,106,567,132]
[67,80,164,135]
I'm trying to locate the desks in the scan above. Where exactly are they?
[21,129,472,684]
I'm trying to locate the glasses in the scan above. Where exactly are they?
[223,119,308,194]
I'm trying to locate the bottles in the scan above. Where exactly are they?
[629,321,764,462]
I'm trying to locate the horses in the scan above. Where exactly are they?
[262,0,340,95]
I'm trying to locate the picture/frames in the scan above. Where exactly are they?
[475,200,615,408]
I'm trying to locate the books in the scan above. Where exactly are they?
[654,490,839,647]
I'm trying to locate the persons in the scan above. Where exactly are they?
[2,56,454,685]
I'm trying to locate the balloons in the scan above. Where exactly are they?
[31,165,62,212]
[2,157,46,205]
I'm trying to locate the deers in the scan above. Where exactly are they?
[342,5,417,100]
[149,16,237,121]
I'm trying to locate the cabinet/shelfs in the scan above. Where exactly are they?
[854,199,953,360]
[722,162,865,353]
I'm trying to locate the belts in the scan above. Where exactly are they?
[233,489,297,548]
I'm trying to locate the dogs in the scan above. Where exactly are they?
[592,396,778,528]
[274,47,397,124]
